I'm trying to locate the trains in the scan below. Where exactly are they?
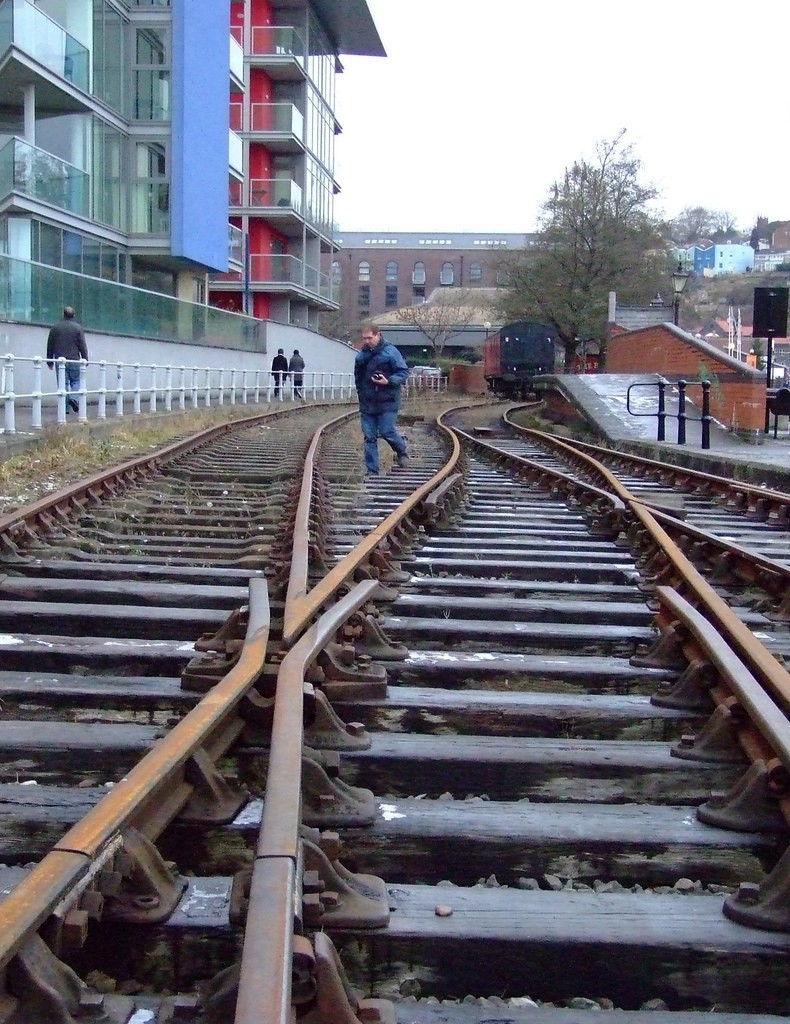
[483,322,555,400]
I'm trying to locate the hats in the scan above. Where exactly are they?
[294,350,299,354]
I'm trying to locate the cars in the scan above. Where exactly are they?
[406,366,442,387]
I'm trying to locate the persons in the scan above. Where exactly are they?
[271,348,288,397]
[289,350,306,397]
[352,324,409,474]
[47,306,89,414]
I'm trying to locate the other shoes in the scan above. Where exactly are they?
[69,399,79,413]
[297,392,302,398]
[397,449,409,468]
[364,469,378,476]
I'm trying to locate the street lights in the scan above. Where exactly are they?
[671,261,689,326]
[484,322,491,339]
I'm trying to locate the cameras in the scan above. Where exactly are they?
[373,371,382,381]
